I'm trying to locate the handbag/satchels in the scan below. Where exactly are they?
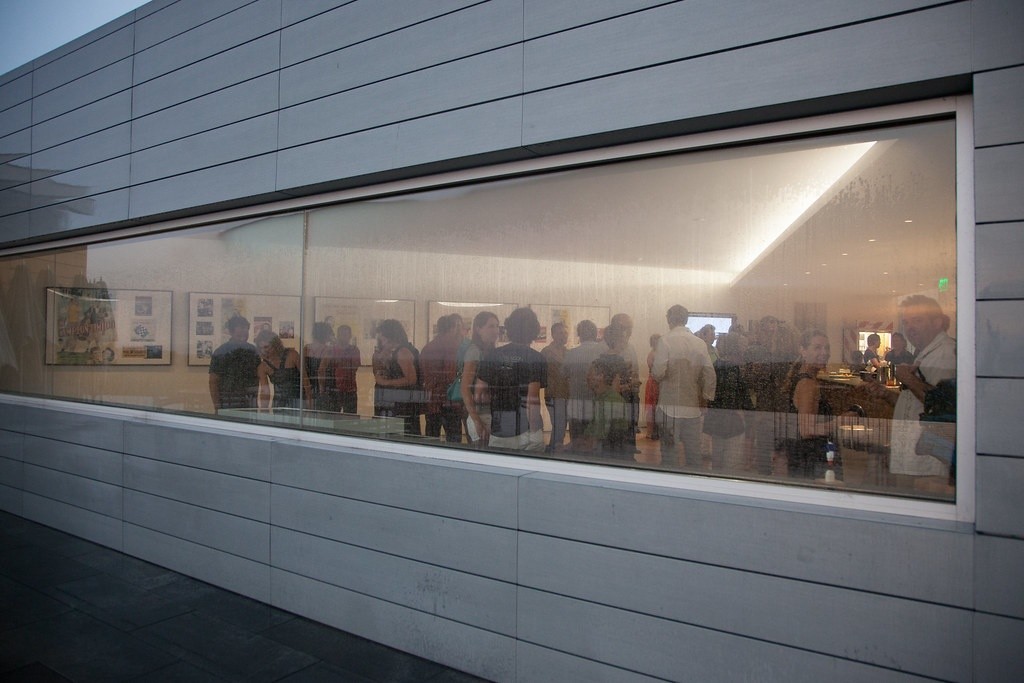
[917,378,957,464]
[785,437,843,482]
[395,385,429,415]
[644,377,659,406]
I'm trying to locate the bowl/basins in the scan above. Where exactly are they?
[859,371,877,382]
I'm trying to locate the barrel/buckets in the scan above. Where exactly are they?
[890,363,903,385]
[877,366,890,384]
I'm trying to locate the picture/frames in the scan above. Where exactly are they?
[44,287,611,368]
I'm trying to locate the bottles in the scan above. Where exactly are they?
[860,359,866,371]
[826,442,835,467]
[867,358,891,371]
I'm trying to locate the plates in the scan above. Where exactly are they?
[885,385,900,389]
[834,379,850,381]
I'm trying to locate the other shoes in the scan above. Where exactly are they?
[645,433,658,439]
[634,449,642,455]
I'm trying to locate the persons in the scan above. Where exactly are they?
[420,313,472,442]
[604,312,642,453]
[372,319,420,436]
[889,295,956,497]
[787,330,858,482]
[331,326,361,413]
[885,333,913,363]
[645,305,716,470]
[572,353,634,458]
[257,329,313,411]
[474,307,549,453]
[209,318,262,416]
[851,347,863,369]
[541,322,571,450]
[703,316,787,476]
[564,319,606,446]
[864,334,882,366]
[89,345,114,363]
[460,311,500,439]
[303,322,336,411]
